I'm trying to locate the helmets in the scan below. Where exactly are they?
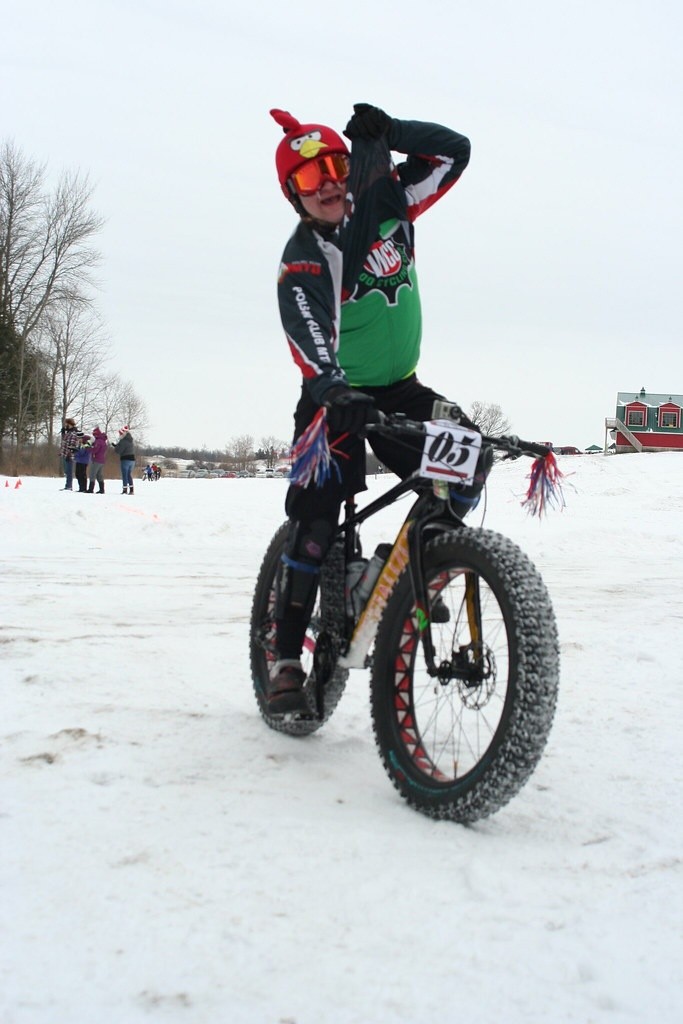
[268,108,352,200]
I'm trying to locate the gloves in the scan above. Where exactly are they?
[324,391,378,439]
[343,103,395,147]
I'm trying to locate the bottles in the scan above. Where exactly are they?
[346,552,370,618]
[358,543,394,603]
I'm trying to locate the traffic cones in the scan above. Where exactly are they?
[5,480,9,487]
[18,478,22,485]
[14,481,19,489]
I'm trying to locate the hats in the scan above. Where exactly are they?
[93,428,101,436]
[66,418,75,426]
[82,435,90,443]
[119,426,128,435]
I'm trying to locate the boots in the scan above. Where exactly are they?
[84,482,95,493]
[97,483,104,493]
[122,486,127,494]
[128,485,134,495]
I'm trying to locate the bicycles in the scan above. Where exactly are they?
[141,469,160,481]
[247,405,560,827]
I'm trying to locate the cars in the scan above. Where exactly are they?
[164,466,292,479]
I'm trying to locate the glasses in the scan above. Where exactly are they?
[290,154,352,196]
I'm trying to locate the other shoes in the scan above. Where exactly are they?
[267,670,308,710]
[430,597,448,623]
[60,487,71,491]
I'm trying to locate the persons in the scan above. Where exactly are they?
[69,431,91,492]
[265,102,493,714]
[143,464,162,481]
[112,426,135,494]
[57,419,79,490]
[84,428,108,493]
